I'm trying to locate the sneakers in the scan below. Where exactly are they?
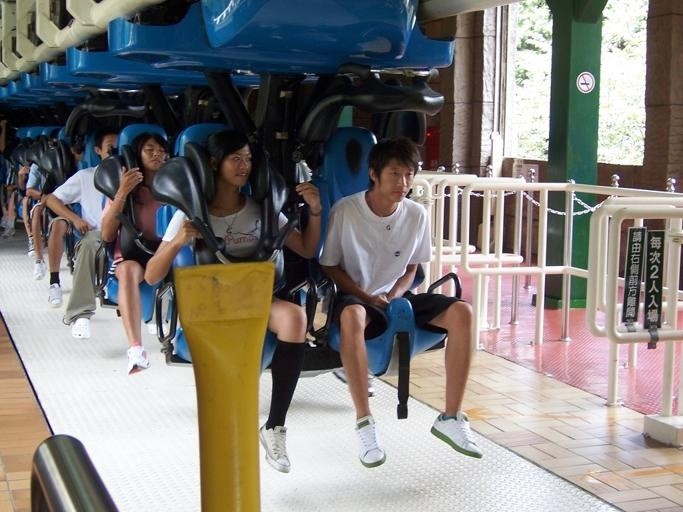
[429,412,485,461]
[47,283,62,307]
[258,424,293,474]
[125,344,150,376]
[26,235,36,257]
[353,414,386,468]
[0,216,15,239]
[33,260,47,280]
[71,317,91,340]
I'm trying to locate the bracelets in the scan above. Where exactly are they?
[310,205,322,217]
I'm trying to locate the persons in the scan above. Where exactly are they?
[143,128,322,473]
[320,136,481,469]
